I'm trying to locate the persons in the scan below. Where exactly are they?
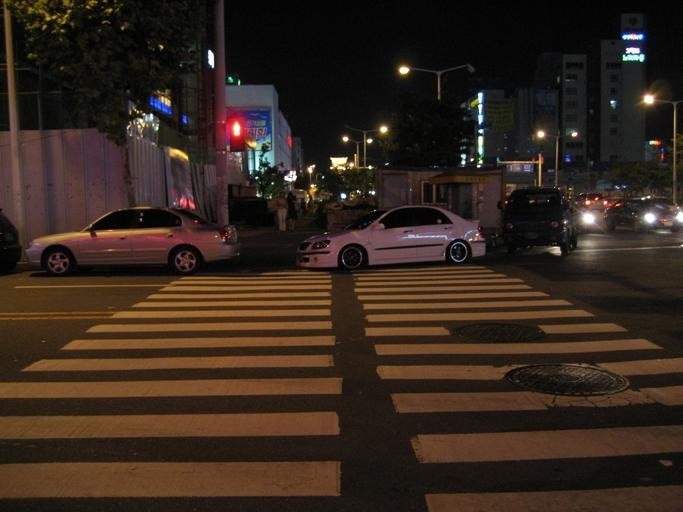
[308,197,315,215]
[277,192,298,231]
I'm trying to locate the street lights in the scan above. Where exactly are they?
[342,124,387,168]
[645,96,683,205]
[399,64,475,100]
[538,131,578,186]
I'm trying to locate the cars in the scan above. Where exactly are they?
[295,204,486,270]
[24,206,240,277]
[576,192,606,212]
[497,188,578,254]
[604,198,682,233]
[0,208,21,273]
